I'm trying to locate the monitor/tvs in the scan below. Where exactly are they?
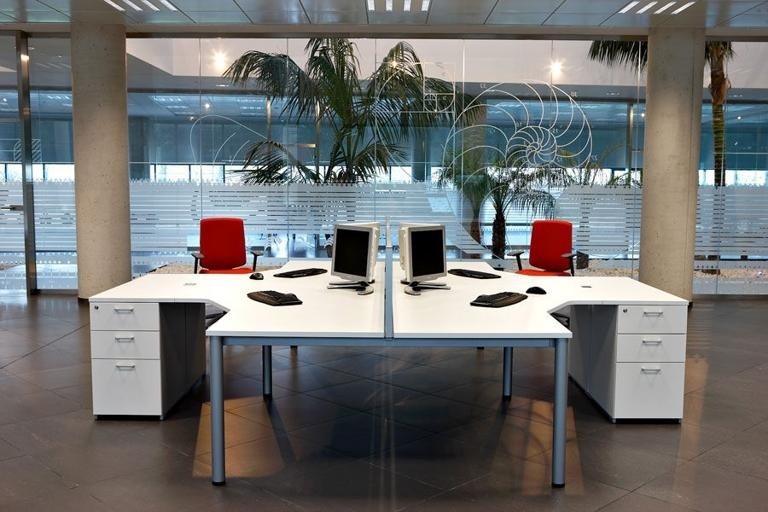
[327,221,380,295]
[399,222,451,296]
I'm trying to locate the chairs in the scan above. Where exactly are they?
[191,218,264,274]
[507,220,581,276]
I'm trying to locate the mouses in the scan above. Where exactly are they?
[251,272,263,280]
[475,294,493,303]
[527,287,547,294]
[279,293,298,301]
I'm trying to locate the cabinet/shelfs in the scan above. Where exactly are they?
[90,300,206,421]
[569,303,687,427]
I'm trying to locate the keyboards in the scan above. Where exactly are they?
[448,269,502,279]
[274,268,328,278]
[247,289,303,306]
[470,291,528,307]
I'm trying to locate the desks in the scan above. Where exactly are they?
[90,261,391,487]
[388,261,691,489]
